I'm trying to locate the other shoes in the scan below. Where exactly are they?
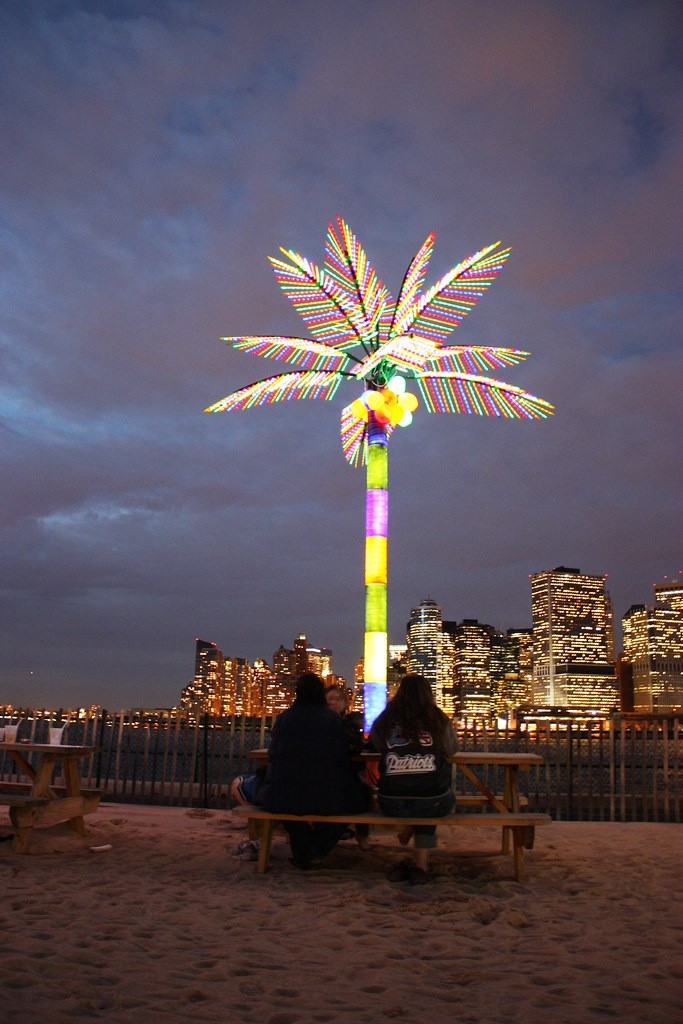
[288,854,320,870]
[397,833,409,846]
[409,867,433,885]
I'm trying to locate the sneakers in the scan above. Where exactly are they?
[228,837,261,860]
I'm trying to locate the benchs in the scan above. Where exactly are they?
[0,781,106,796]
[454,793,527,807]
[232,807,552,826]
[0,794,50,808]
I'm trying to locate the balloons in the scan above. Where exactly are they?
[352,375,418,427]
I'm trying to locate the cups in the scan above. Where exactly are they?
[0,728,5,741]
[4,725,19,743]
[50,728,63,745]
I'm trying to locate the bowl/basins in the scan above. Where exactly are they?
[20,739,30,743]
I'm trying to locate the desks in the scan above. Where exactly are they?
[0,742,103,853]
[247,748,545,883]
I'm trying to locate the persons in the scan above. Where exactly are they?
[232,673,374,872]
[367,675,461,885]
